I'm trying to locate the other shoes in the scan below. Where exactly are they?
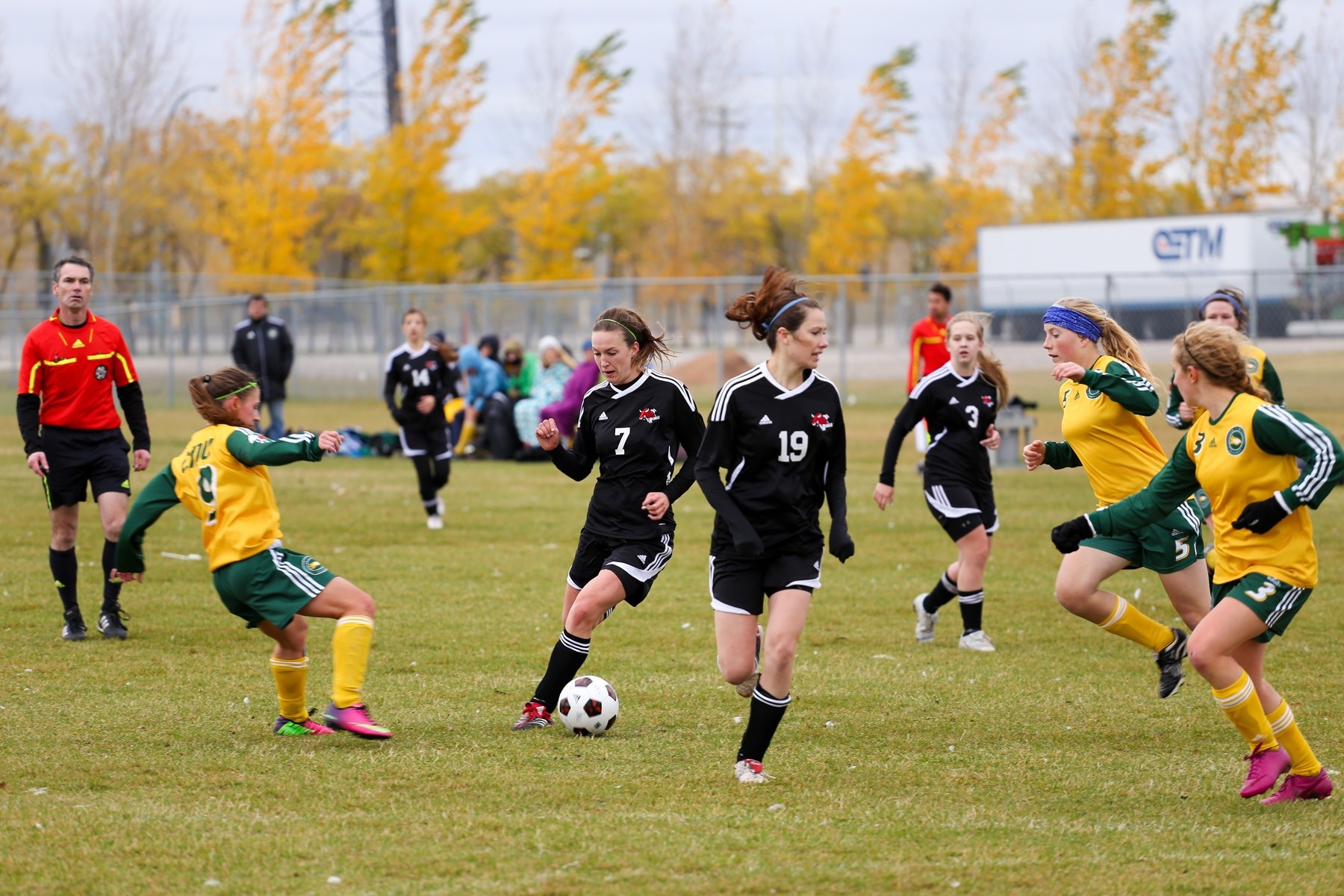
[447,448,551,464]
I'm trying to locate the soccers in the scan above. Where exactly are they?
[557,675,620,738]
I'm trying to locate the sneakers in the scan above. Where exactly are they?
[96,601,128,638]
[272,715,334,735]
[322,702,391,738]
[437,499,446,516]
[918,465,924,474]
[736,625,762,697]
[1154,626,1191,698]
[732,759,768,785]
[1240,745,1292,799]
[959,629,995,652]
[511,697,556,729]
[1260,768,1333,805]
[912,593,937,646]
[426,516,443,529]
[62,607,87,641]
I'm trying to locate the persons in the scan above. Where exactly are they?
[12,260,151,645]
[905,281,964,476]
[1023,296,1217,699]
[516,304,707,734]
[232,294,294,443]
[692,267,859,789]
[1051,324,1343,804]
[872,312,1008,653]
[1162,289,1288,431]
[382,309,454,529]
[417,327,603,457]
[104,369,402,744]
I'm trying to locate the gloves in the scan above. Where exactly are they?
[1051,516,1093,554]
[1231,497,1289,535]
